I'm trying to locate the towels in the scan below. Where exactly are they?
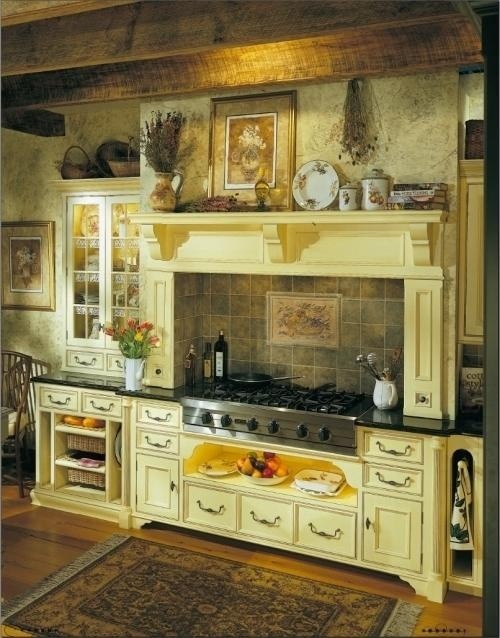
[448,456,476,552]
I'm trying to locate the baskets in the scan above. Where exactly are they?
[61,137,140,179]
[67,469,105,488]
[67,435,105,455]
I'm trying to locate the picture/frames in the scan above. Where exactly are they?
[2,218,57,313]
[206,88,298,213]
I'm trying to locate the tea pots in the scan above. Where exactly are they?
[123,357,146,392]
[372,377,398,411]
[150,171,183,212]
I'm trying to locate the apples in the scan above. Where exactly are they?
[264,452,289,478]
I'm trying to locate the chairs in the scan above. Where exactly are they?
[2,348,33,498]
[4,358,52,415]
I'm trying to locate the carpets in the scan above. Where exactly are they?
[0,531,430,637]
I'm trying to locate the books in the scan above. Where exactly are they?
[387,183,448,210]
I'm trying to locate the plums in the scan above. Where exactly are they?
[250,456,271,476]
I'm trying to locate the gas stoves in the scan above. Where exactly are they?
[179,384,374,458]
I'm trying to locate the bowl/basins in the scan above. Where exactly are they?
[236,464,291,487]
[106,159,140,178]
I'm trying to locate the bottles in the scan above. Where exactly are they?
[182,343,196,386]
[202,343,212,381]
[338,184,361,211]
[362,170,389,209]
[212,331,228,380]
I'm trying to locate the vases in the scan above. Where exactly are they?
[147,171,186,212]
[127,358,147,391]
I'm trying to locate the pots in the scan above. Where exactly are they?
[227,372,306,383]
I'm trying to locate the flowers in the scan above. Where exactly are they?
[123,106,192,174]
[95,317,161,357]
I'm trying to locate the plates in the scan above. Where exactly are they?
[95,141,137,177]
[292,469,347,498]
[80,204,125,237]
[290,160,339,209]
[198,458,239,477]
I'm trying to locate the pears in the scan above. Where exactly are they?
[238,457,261,478]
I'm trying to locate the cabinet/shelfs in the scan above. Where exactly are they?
[182,476,358,567]
[26,369,128,530]
[119,385,182,530]
[143,270,199,390]
[456,157,484,348]
[402,279,446,420]
[60,176,146,379]
[355,402,443,591]
